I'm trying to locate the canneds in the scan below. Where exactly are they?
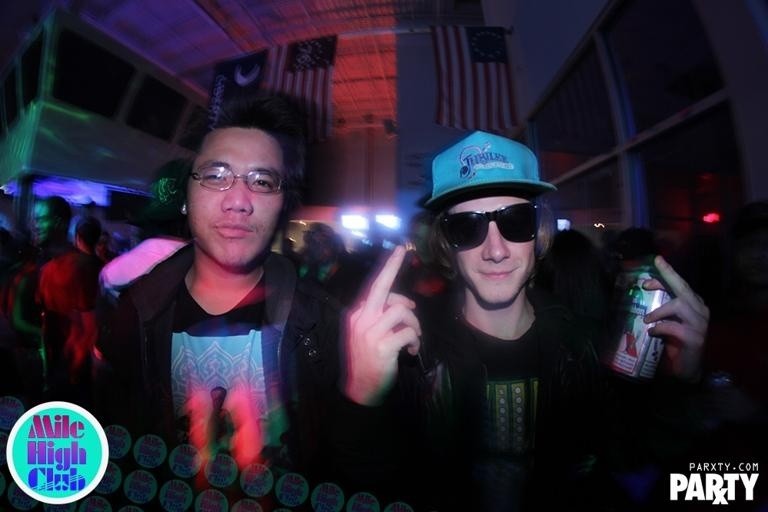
[612,264,675,381]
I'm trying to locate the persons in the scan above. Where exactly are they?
[2,85,767,510]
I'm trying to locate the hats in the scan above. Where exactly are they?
[423,128,559,207]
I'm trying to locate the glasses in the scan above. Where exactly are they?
[188,165,286,195]
[438,199,539,253]
